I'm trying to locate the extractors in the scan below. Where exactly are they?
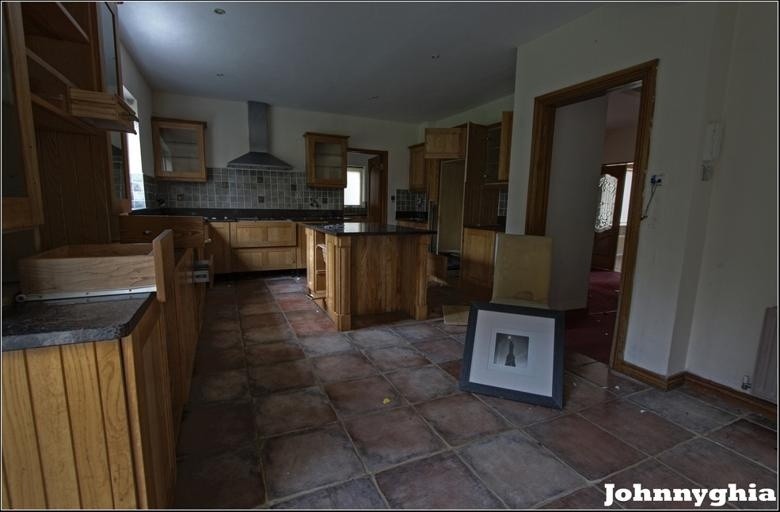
[226,100,294,171]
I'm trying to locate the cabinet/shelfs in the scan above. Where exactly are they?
[407,110,513,289]
[303,133,350,188]
[206,221,438,332]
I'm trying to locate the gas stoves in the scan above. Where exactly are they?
[233,215,286,220]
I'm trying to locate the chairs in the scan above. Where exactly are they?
[489,231,553,311]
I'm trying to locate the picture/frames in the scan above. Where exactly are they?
[457,300,568,411]
